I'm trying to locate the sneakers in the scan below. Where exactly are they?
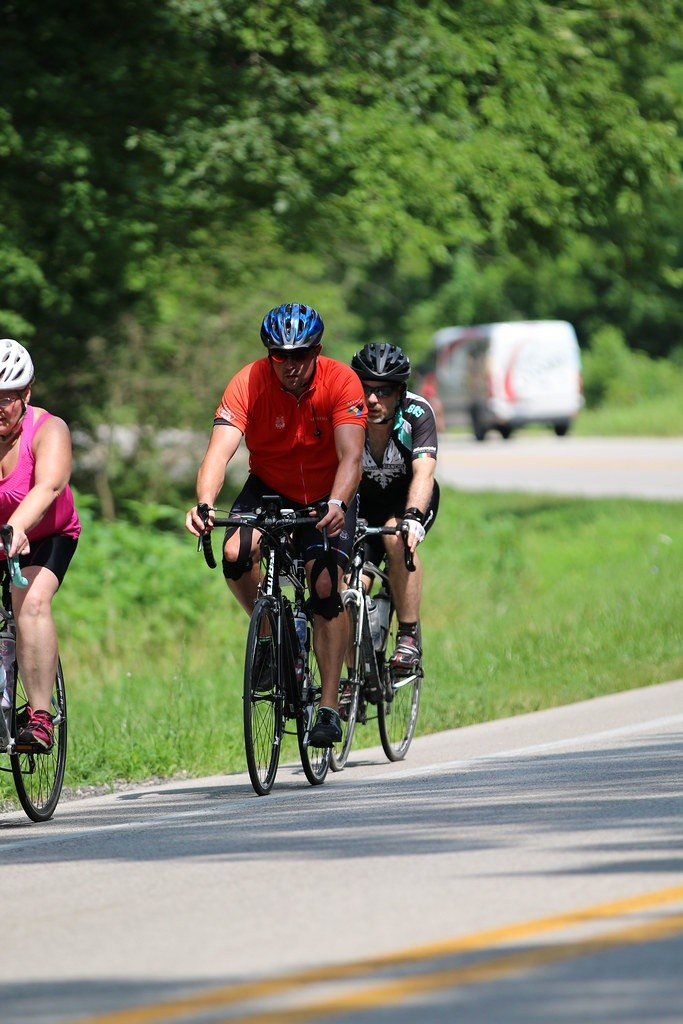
[15,706,55,754]
[308,709,342,748]
[251,640,279,696]
[338,680,365,722]
[388,634,420,669]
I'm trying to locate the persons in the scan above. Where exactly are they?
[186,303,369,742]
[419,371,445,433]
[338,343,439,706]
[0,338,80,751]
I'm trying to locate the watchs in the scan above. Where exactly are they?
[328,500,347,513]
[405,508,423,519]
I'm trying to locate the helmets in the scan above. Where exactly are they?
[0,338,34,390]
[261,303,327,354]
[350,343,411,382]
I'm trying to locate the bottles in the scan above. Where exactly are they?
[282,595,300,660]
[292,612,307,682]
[373,588,390,652]
[365,595,381,650]
[0,632,15,708]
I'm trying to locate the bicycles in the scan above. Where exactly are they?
[1,525,69,823]
[188,497,366,794]
[314,520,426,773]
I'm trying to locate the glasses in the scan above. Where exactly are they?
[1,392,25,407]
[362,385,399,397]
[270,348,316,363]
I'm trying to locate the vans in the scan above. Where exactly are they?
[436,319,583,441]
